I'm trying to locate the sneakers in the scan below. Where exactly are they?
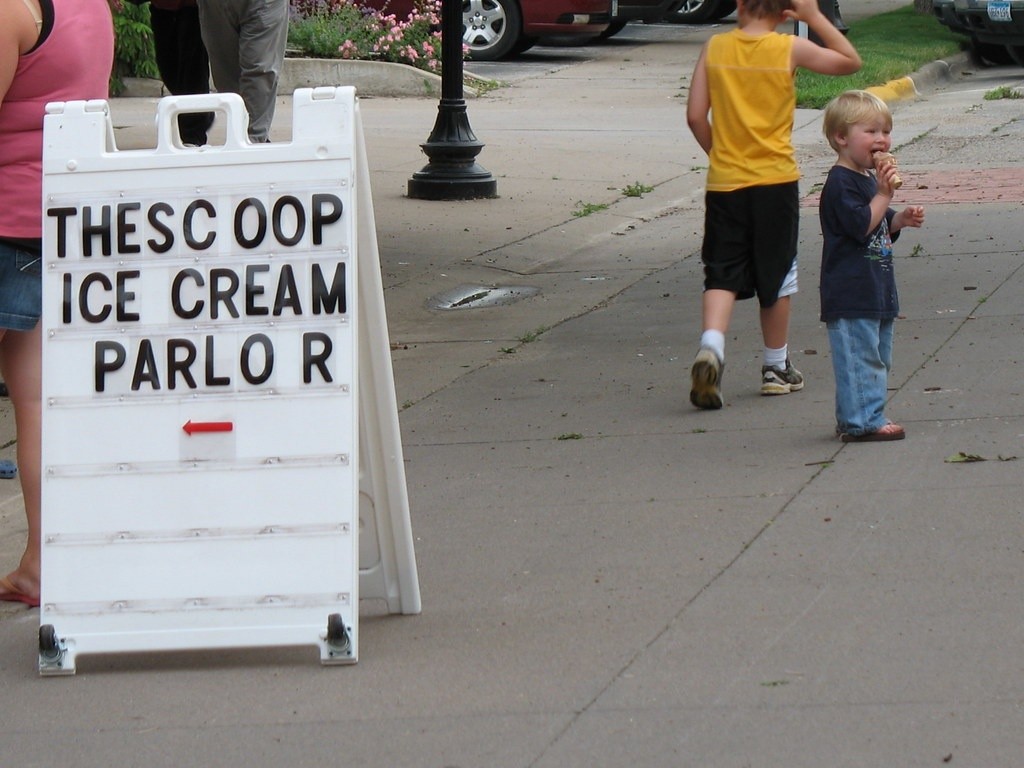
[689,347,724,409]
[758,356,803,395]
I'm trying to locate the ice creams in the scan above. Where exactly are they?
[872,150,903,191]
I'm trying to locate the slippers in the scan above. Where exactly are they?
[0,574,41,605]
[836,423,906,441]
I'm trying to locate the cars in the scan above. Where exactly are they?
[294,0,626,62]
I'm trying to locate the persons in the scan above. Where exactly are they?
[822,91,925,441]
[684,0,863,409]
[196,0,293,144]
[150,0,218,150]
[0,1,114,606]
